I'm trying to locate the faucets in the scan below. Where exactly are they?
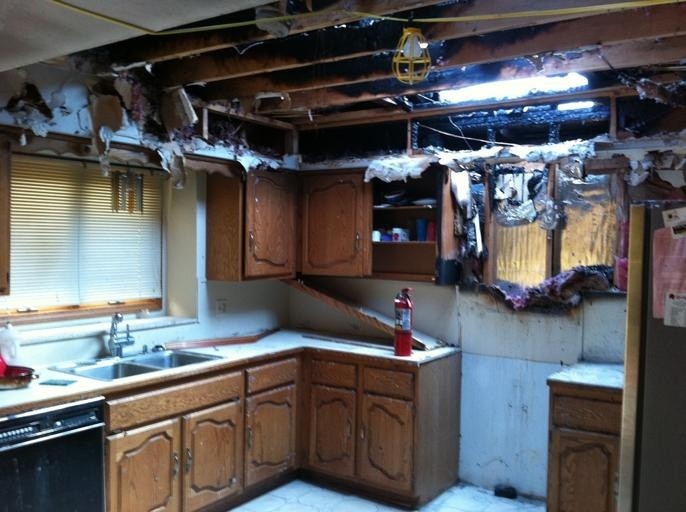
[108,311,124,356]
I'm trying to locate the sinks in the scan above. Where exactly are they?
[74,362,157,380]
[134,352,213,369]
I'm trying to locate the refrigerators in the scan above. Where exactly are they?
[614,198,686,512]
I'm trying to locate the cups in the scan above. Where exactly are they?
[415,216,435,241]
[371,230,382,242]
[391,228,402,242]
[400,229,409,241]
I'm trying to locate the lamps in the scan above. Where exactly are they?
[391,8,432,85]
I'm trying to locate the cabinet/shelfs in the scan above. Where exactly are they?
[206,171,296,284]
[296,170,371,278]
[103,371,244,511]
[371,165,468,283]
[542,387,623,511]
[243,353,302,487]
[303,351,461,510]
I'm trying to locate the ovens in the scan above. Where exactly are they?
[0,393,107,512]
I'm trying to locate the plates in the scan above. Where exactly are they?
[388,197,410,205]
[412,199,438,206]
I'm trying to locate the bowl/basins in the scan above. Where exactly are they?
[0,366,32,390]
[383,189,406,202]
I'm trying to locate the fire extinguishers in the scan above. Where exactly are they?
[393,287,414,356]
